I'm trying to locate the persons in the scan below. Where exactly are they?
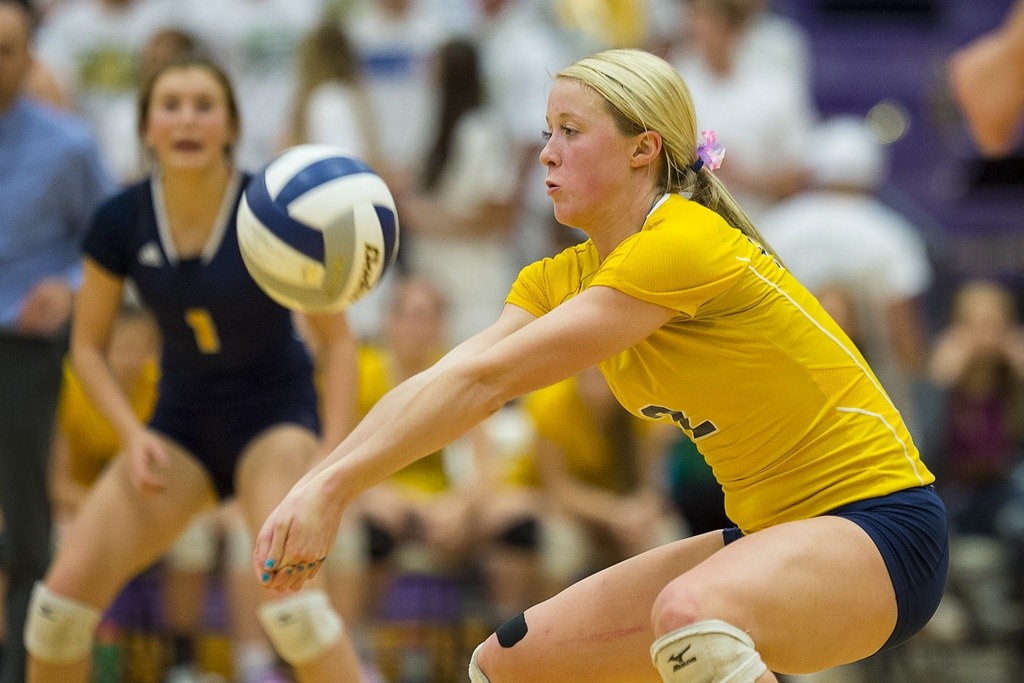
[255,48,948,682]
[1,0,1024,682]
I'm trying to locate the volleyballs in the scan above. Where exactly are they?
[238,144,399,314]
